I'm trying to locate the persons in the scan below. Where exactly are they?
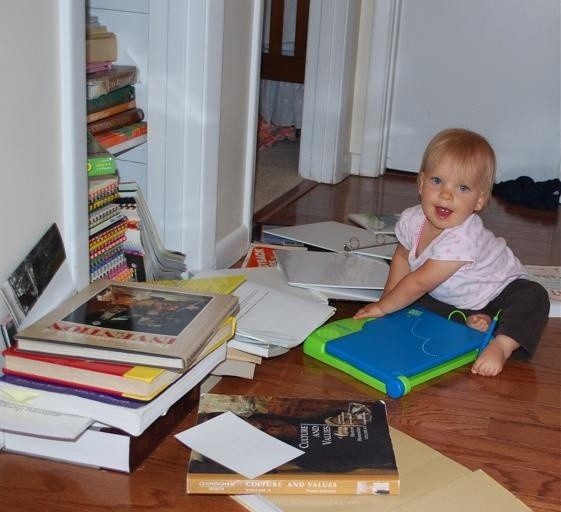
[353,129,550,377]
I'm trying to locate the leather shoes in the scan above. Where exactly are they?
[542,176,561,211]
[493,176,542,209]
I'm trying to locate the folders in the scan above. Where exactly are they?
[262,220,398,255]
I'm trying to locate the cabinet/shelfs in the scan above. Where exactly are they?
[0,0,166,380]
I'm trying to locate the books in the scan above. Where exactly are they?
[348,210,403,235]
[120,178,187,283]
[187,389,401,497]
[522,263,560,318]
[0,276,248,474]
[207,267,337,381]
[86,13,148,150]
[86,132,116,176]
[243,222,305,269]
[90,176,136,286]
